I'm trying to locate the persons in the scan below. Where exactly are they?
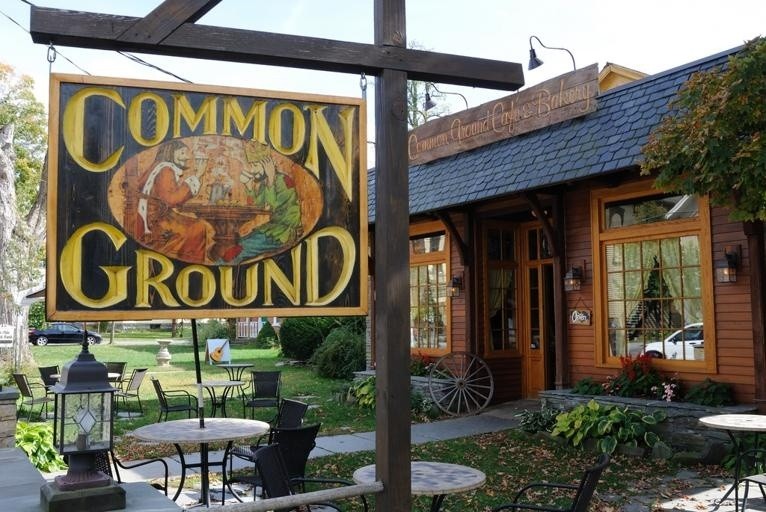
[124,139,300,269]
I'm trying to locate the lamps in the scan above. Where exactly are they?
[37,330,133,511]
[527,34,578,73]
[421,81,472,112]
[712,241,745,285]
[444,270,467,298]
[562,258,586,292]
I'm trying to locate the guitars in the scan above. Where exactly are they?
[210,339,229,362]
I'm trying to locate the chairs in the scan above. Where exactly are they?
[254,442,368,512]
[709,446,766,512]
[486,451,614,512]
[114,368,149,418]
[245,371,281,420]
[111,452,168,496]
[104,362,127,404]
[39,366,61,419]
[13,374,50,421]
[225,398,321,512]
[150,376,198,423]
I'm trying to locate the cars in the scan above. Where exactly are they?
[643,322,705,363]
[28,324,104,347]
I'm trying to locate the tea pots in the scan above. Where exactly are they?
[205,181,231,205]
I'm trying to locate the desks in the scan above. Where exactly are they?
[216,364,254,399]
[350,459,488,511]
[185,381,245,418]
[51,372,120,378]
[135,418,270,508]
[699,412,766,512]
